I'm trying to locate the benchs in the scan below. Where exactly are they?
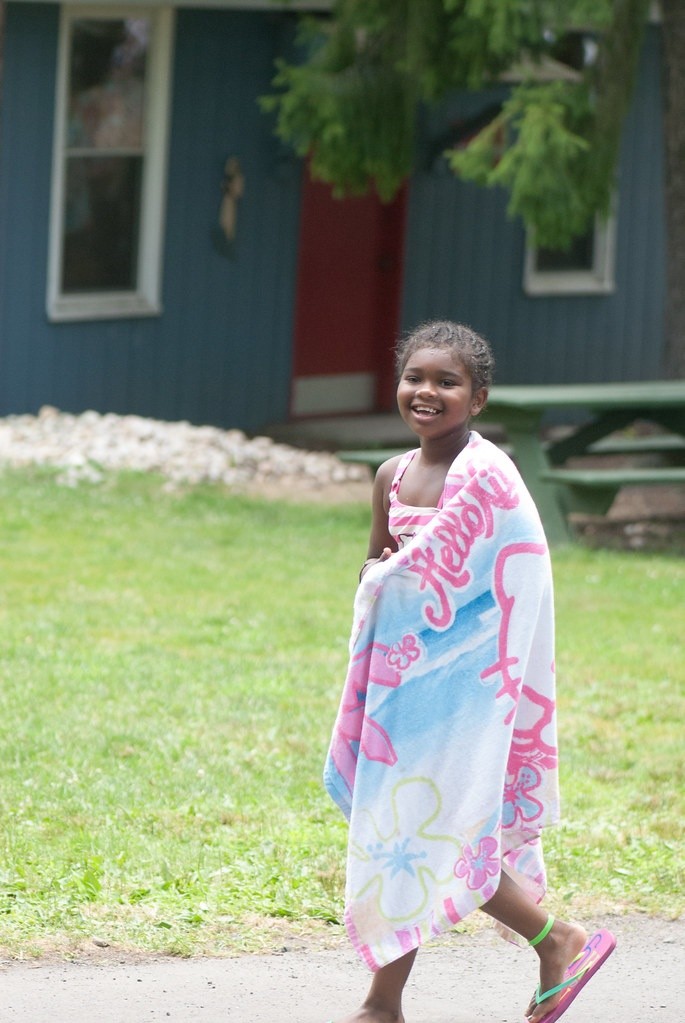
[345,437,684,546]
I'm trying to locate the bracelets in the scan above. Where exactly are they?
[358,563,370,584]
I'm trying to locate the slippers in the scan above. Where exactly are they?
[527,928,617,1023]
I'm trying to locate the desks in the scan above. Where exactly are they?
[479,379,684,469]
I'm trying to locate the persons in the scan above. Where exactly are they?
[327,321,617,1023]
